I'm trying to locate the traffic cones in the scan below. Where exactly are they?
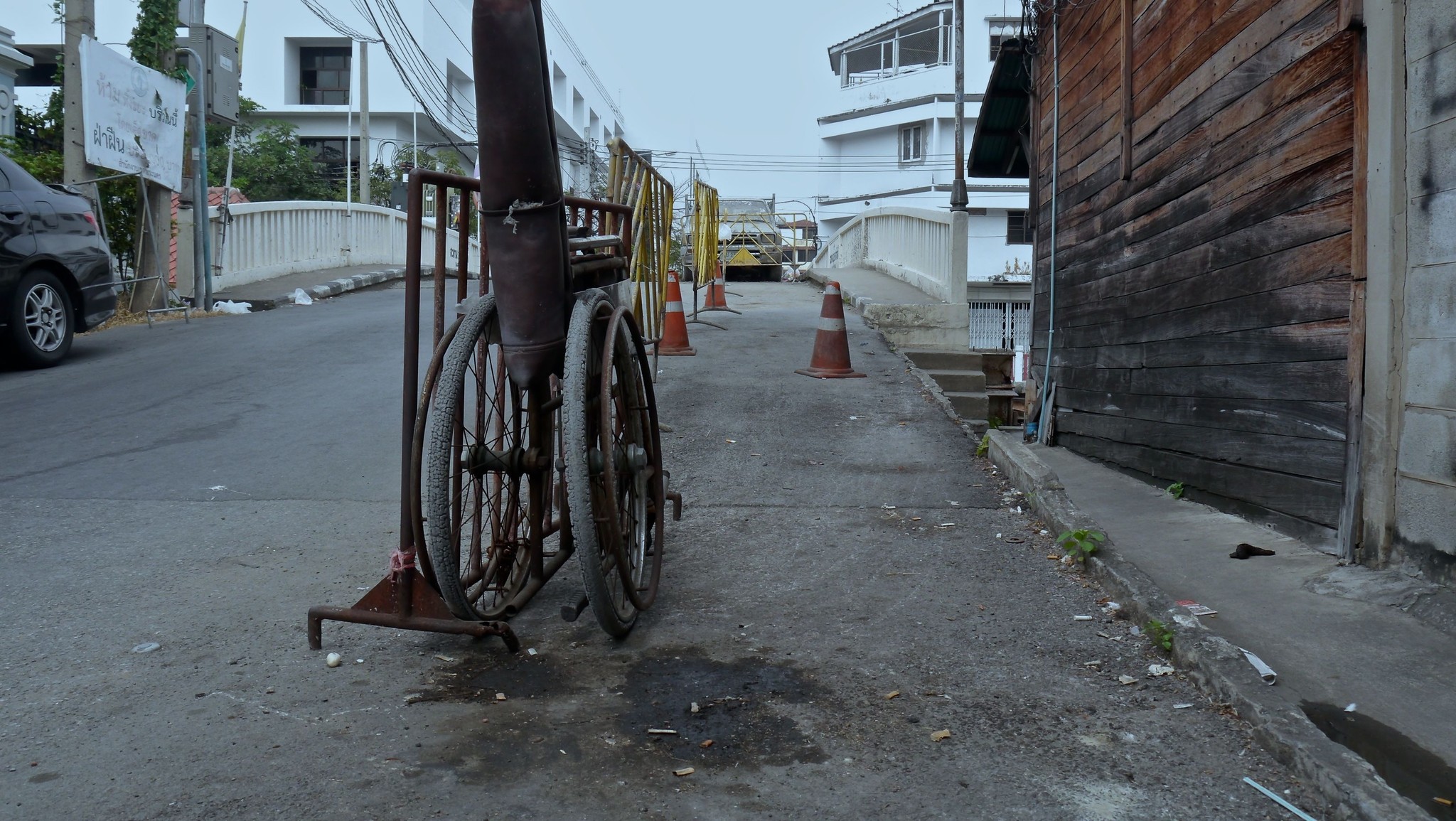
[642,270,697,356]
[793,280,868,379]
[700,258,731,311]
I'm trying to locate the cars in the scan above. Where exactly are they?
[0,150,117,367]
[680,197,784,282]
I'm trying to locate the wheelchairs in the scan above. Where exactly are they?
[420,226,681,637]
